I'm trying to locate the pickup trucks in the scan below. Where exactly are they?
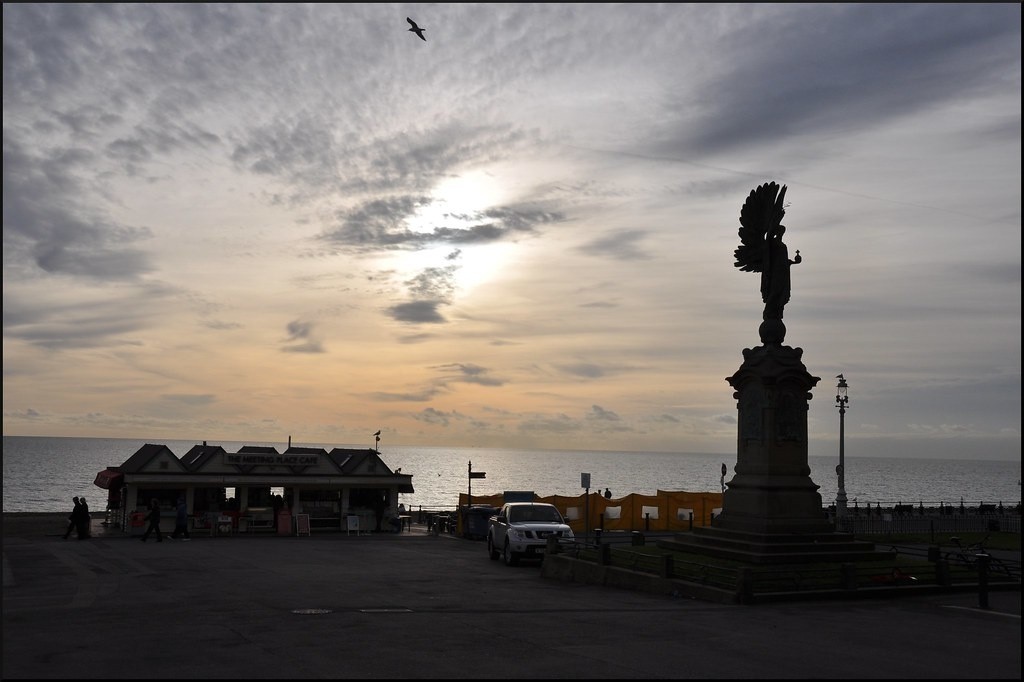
[487,503,575,565]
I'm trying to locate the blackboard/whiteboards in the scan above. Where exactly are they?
[348,516,359,529]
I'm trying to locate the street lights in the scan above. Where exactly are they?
[719,464,726,512]
[834,375,850,518]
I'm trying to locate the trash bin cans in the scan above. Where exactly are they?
[426,512,440,532]
[277,510,291,535]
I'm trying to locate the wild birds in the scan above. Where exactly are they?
[406,17,427,42]
[373,430,381,436]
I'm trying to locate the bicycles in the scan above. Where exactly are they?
[945,534,1011,578]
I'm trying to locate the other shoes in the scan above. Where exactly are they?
[156,539,162,542]
[140,538,146,542]
[61,536,67,539]
[167,535,174,541]
[181,538,191,542]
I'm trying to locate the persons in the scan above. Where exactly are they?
[399,504,406,512]
[167,496,191,541]
[760,202,801,322]
[61,497,88,540]
[140,498,162,542]
[605,488,611,499]
